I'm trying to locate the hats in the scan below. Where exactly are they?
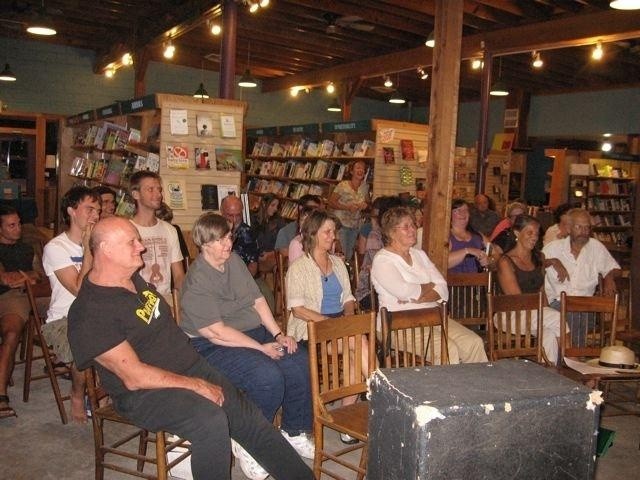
[586,344,640,374]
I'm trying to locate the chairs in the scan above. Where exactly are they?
[447,270,488,343]
[307,310,376,480]
[558,291,640,418]
[85,366,192,480]
[23,280,88,424]
[597,273,640,357]
[488,292,555,371]
[380,300,448,369]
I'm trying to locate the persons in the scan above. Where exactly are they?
[67,217,317,479]
[275,194,321,257]
[541,210,622,360]
[287,206,315,264]
[359,202,370,253]
[284,209,382,445]
[40,185,102,424]
[543,204,580,248]
[154,202,189,271]
[90,186,116,218]
[251,192,287,316]
[355,198,401,317]
[468,193,501,236]
[370,207,490,366]
[494,216,571,365]
[221,196,276,317]
[446,199,488,278]
[331,159,369,261]
[0,204,40,418]
[126,171,185,320]
[179,213,315,479]
[490,204,526,267]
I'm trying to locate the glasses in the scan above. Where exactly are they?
[568,222,591,231]
[388,223,418,230]
[211,233,236,247]
[451,209,470,215]
[507,214,518,221]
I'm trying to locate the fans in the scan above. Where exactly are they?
[296,13,375,32]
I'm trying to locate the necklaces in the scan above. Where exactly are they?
[313,254,328,282]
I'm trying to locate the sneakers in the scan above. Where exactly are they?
[229,434,271,479]
[339,432,361,446]
[276,425,316,460]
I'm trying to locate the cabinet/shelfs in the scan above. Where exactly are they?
[569,158,640,267]
[1,109,61,298]
[452,145,527,217]
[541,149,602,209]
[245,120,427,222]
[61,92,248,232]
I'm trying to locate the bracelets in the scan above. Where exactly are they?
[465,248,470,255]
[274,332,282,340]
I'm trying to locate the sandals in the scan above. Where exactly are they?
[42,361,74,375]
[0,393,16,418]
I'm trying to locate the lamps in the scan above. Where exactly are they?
[326,99,342,112]
[26,1,57,36]
[0,32,17,81]
[238,37,258,88]
[415,66,428,80]
[532,50,543,67]
[192,56,209,99]
[490,56,509,97]
[425,32,435,47]
[389,73,406,103]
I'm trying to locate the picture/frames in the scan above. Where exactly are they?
[505,109,518,119]
[504,119,518,128]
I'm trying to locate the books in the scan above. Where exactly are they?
[400,166,413,186]
[196,113,212,136]
[383,146,395,165]
[380,128,395,144]
[251,138,374,158]
[70,122,141,152]
[164,179,186,210]
[70,151,160,186]
[113,188,136,218]
[400,139,415,161]
[585,176,632,246]
[219,112,236,137]
[247,194,298,218]
[166,142,189,169]
[245,177,331,201]
[216,145,243,172]
[415,178,426,199]
[201,184,218,211]
[247,158,373,181]
[417,150,427,167]
[169,109,188,135]
[195,147,211,170]
[217,184,238,212]
